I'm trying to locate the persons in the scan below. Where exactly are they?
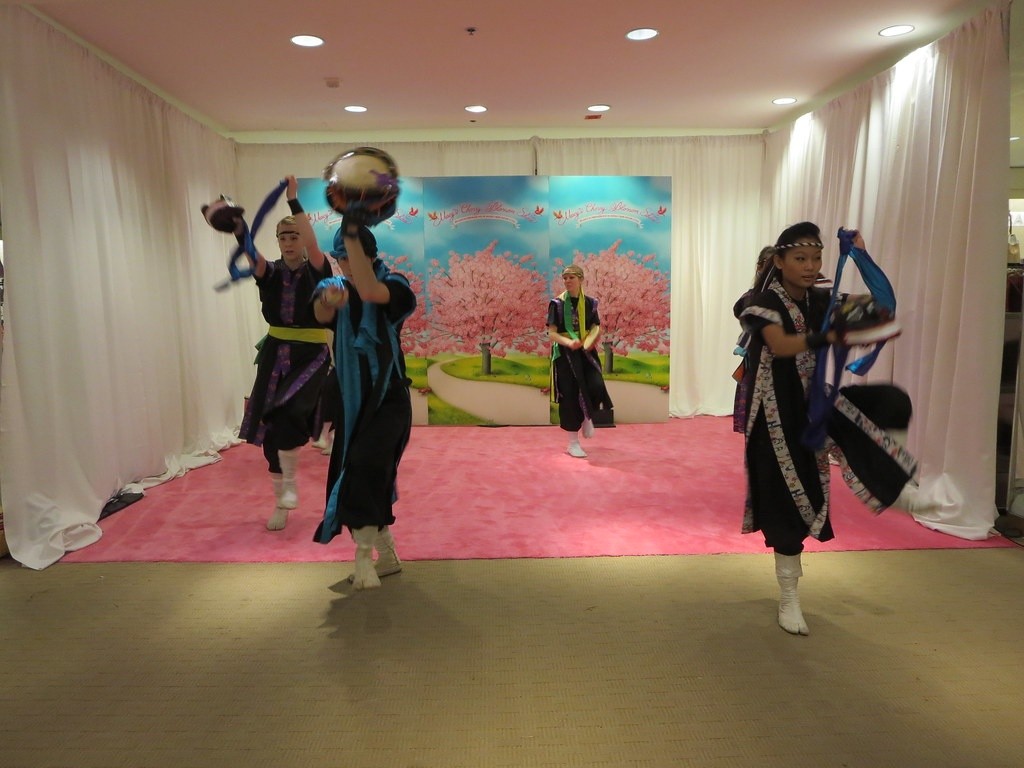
[231,174,334,530]
[308,199,418,589]
[735,222,965,635]
[545,266,616,458]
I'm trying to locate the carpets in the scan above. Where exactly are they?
[53,413,1020,564]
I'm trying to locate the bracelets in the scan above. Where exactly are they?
[287,199,304,215]
[807,333,830,349]
